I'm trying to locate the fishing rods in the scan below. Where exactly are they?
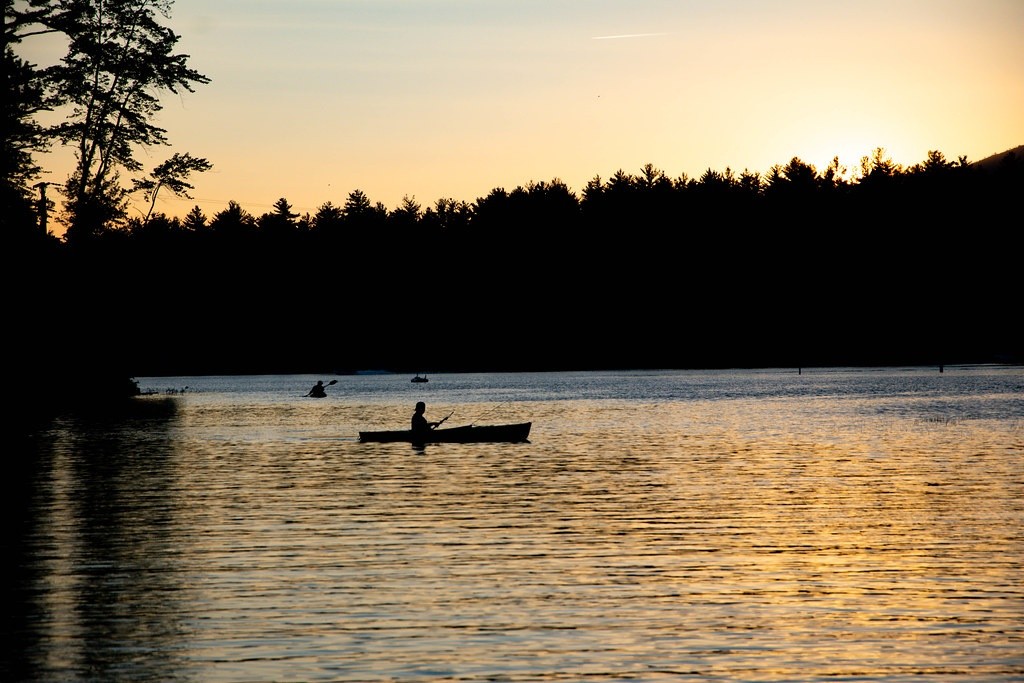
[470,397,513,425]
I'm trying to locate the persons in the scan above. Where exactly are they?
[309,381,326,398]
[410,401,439,433]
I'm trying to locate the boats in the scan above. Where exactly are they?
[311,393,326,398]
[358,420,533,443]
[411,375,428,383]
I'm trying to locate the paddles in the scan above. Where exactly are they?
[303,379,339,398]
[433,411,455,430]
